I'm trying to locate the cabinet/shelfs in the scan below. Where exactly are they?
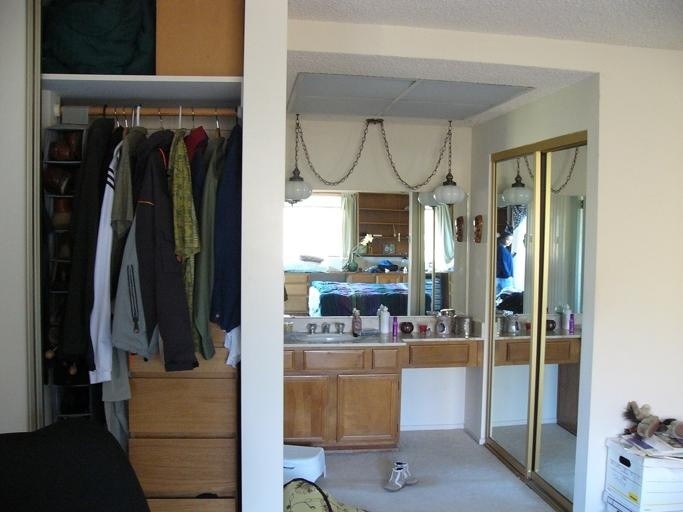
[354,191,410,258]
[312,270,408,286]
[283,341,483,452]
[489,333,532,368]
[28,0,243,510]
[543,332,581,367]
[286,269,310,315]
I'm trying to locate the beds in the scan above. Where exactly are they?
[309,281,411,316]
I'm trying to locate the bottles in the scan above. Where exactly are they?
[378,303,389,334]
[392,317,398,336]
[400,255,407,271]
[376,304,383,316]
[351,310,362,337]
[546,319,555,331]
[351,308,356,316]
[425,328,431,336]
[561,304,572,329]
[495,308,531,338]
[452,315,475,338]
[435,309,459,337]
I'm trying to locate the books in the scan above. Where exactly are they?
[618,431,683,461]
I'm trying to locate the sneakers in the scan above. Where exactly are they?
[393,461,418,486]
[383,466,407,491]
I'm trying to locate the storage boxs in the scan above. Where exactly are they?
[117,299,240,510]
[156,0,245,77]
[602,436,682,511]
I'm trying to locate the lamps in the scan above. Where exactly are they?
[431,116,468,207]
[285,112,312,205]
[502,157,532,206]
[417,188,440,209]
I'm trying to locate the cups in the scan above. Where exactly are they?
[419,325,427,336]
[284,322,293,335]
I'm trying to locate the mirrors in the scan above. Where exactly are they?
[487,143,532,473]
[285,189,456,319]
[532,128,588,510]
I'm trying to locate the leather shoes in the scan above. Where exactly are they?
[42,133,82,326]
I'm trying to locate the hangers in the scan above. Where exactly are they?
[96,102,241,158]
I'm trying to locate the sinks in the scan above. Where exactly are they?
[307,333,351,340]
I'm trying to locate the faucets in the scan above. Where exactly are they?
[321,323,330,333]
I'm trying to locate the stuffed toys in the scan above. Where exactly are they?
[623,400,683,441]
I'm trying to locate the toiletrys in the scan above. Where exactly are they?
[392,316,398,336]
